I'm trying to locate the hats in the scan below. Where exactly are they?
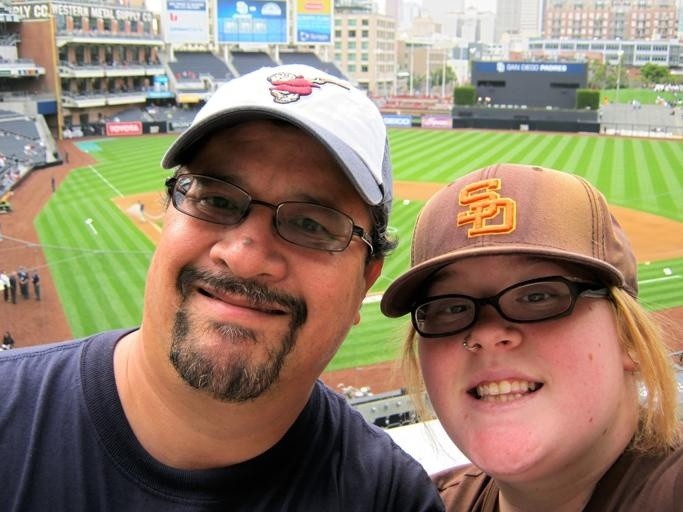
[381,162,638,319]
[161,63,393,206]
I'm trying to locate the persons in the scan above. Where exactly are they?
[176,70,197,81]
[50,175,56,193]
[0,265,41,304]
[2,330,16,350]
[380,163,683,512]
[0,128,39,215]
[0,64,445,511]
[137,200,145,223]
[64,150,69,164]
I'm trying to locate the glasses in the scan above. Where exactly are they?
[411,275,600,338]
[165,172,373,261]
